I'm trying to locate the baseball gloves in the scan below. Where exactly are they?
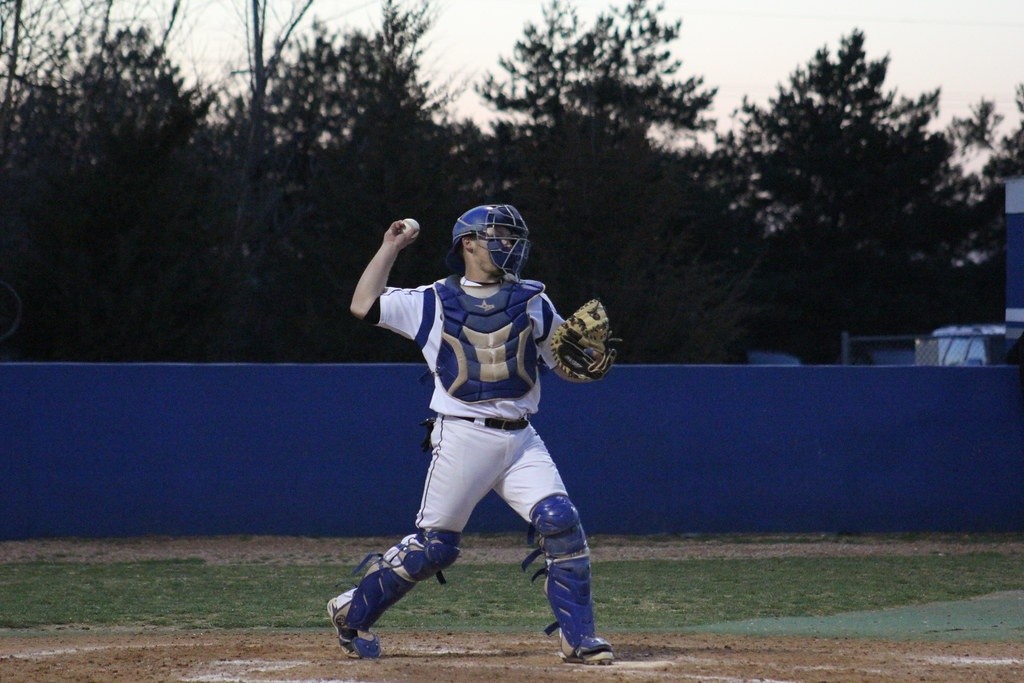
[550,298,624,383]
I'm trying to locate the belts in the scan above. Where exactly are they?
[458,416,529,432]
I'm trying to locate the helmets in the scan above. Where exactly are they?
[445,206,497,272]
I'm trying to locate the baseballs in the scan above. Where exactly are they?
[399,218,420,239]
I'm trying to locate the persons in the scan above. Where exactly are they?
[326,204,616,666]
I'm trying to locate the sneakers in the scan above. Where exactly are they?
[558,632,614,667]
[327,597,364,659]
